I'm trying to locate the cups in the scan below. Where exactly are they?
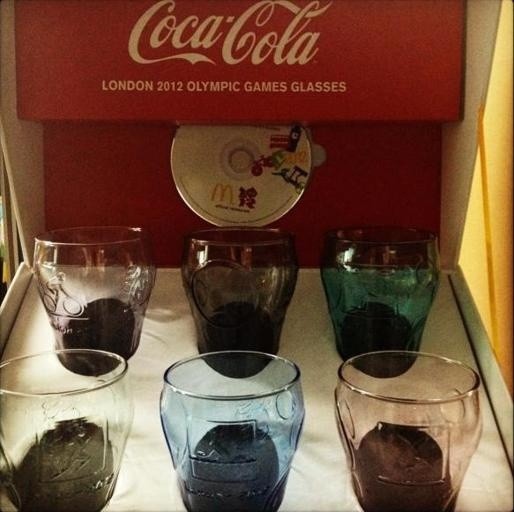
[0,349,133,512]
[319,225,441,378]
[181,228,299,378]
[159,350,305,512]
[32,225,157,376]
[332,350,483,512]
